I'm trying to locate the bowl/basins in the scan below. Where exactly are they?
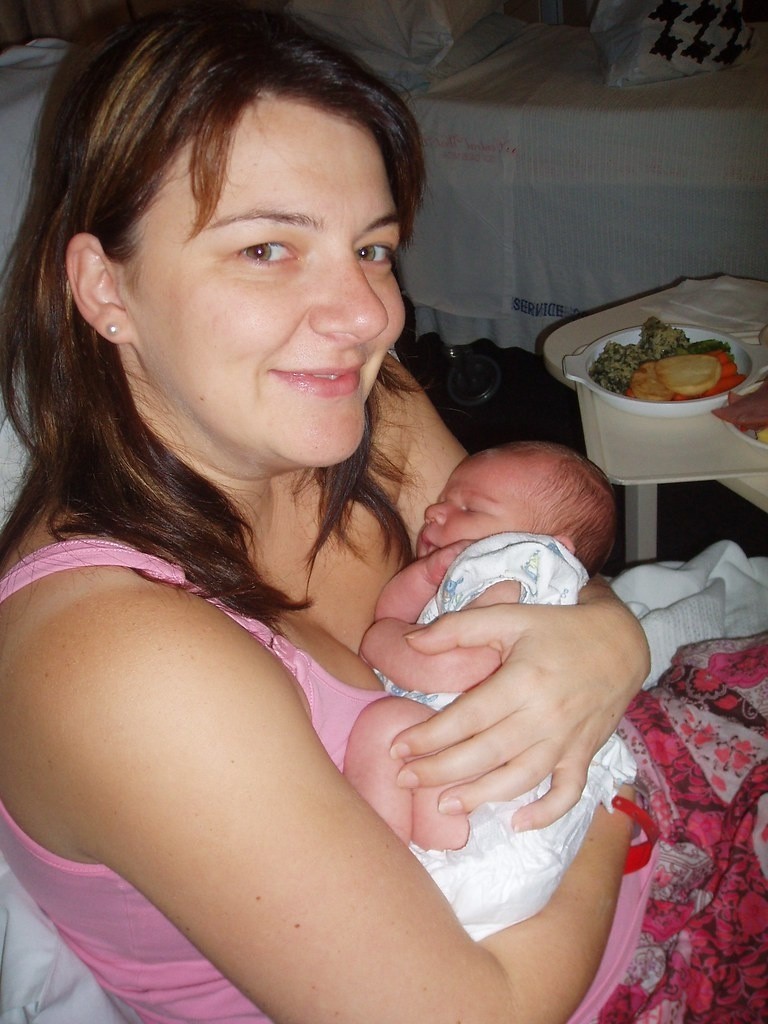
[563,326,758,418]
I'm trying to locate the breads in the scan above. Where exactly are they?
[654,355,722,396]
[630,360,675,401]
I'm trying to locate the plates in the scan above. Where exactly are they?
[722,382,768,449]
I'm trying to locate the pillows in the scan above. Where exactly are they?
[285,0,528,92]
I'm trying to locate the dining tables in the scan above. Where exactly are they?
[544,282,768,570]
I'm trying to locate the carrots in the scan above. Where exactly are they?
[625,350,746,400]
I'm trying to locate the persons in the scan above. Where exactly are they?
[344,439,617,944]
[0,1,662,1022]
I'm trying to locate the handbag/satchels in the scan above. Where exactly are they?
[590,1,761,86]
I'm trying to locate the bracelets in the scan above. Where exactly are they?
[611,796,658,875]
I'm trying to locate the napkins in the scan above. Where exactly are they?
[641,272,767,347]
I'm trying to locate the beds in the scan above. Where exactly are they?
[374,20,768,357]
